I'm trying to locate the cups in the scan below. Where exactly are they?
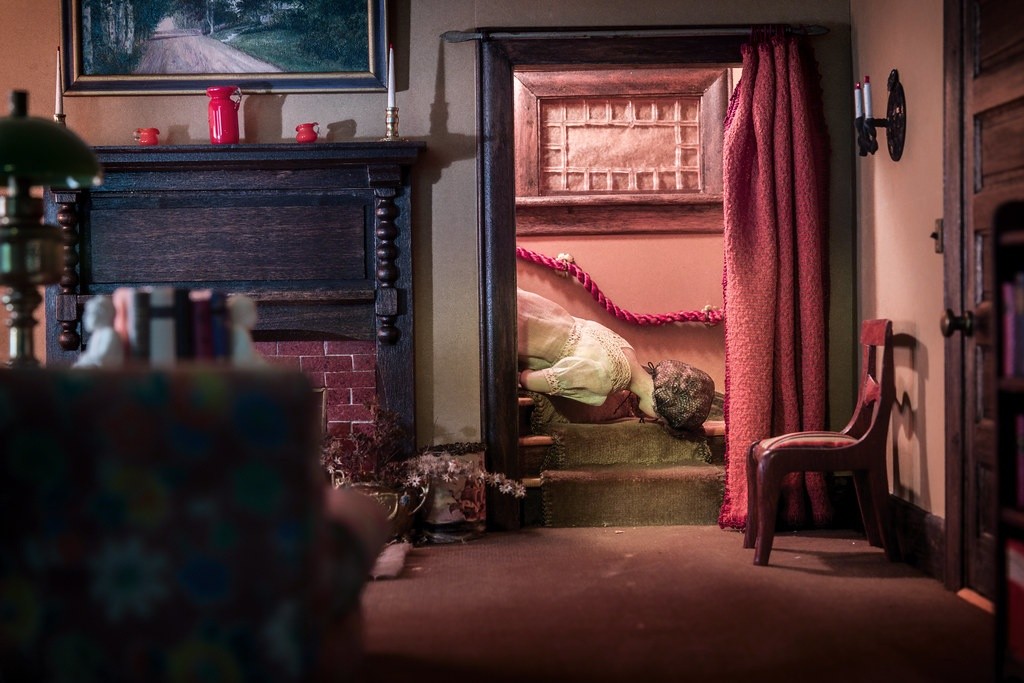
[132,128,160,146]
[114,285,228,371]
[296,122,320,144]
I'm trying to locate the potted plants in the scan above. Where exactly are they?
[318,405,526,540]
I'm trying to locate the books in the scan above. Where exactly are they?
[111,285,232,378]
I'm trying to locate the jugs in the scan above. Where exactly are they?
[206,86,242,145]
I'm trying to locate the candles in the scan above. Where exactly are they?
[55,47,63,116]
[387,45,395,108]
[862,75,874,119]
[855,80,863,118]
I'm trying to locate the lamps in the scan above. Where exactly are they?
[0,88,102,376]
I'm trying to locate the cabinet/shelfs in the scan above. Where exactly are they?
[47,136,429,459]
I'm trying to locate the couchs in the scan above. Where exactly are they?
[0,367,397,683]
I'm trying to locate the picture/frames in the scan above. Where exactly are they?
[58,0,390,98]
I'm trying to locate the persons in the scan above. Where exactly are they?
[69,293,125,373]
[227,292,290,379]
[517,285,716,432]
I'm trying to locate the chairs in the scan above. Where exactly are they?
[741,319,895,566]
